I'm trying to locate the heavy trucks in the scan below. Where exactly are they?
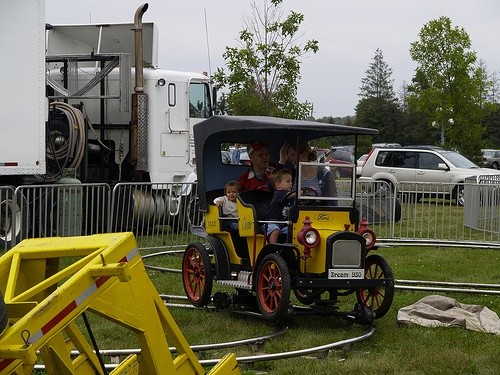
[0,0,217,232]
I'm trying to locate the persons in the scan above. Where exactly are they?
[213,180,240,229]
[236,142,273,192]
[274,137,338,207]
[232,143,242,165]
[261,169,316,244]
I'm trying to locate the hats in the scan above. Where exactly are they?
[247,142,265,157]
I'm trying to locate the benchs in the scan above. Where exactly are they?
[205,187,268,234]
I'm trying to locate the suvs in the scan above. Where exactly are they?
[360,146,495,206]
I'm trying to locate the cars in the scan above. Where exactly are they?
[220,134,499,179]
